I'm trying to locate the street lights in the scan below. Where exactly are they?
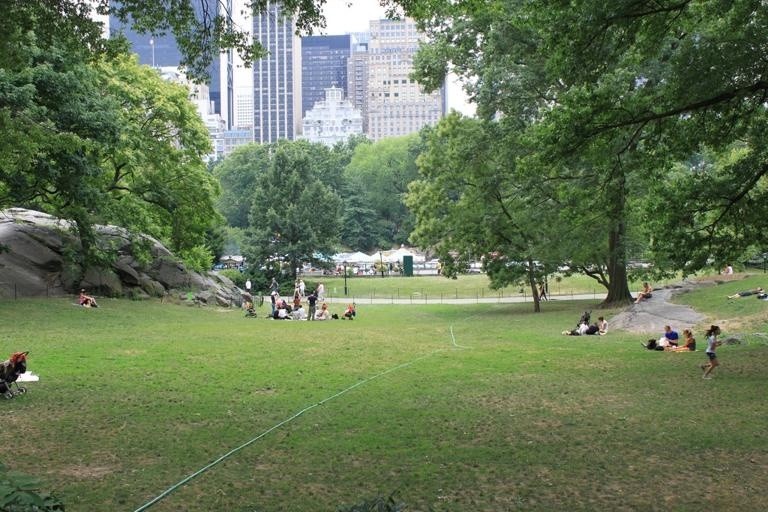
[377,248,384,277]
[342,259,348,295]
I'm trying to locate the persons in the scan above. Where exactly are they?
[562,321,590,336]
[673,332,696,352]
[699,324,723,381]
[322,258,404,278]
[665,329,691,350]
[538,281,549,302]
[242,275,358,321]
[217,259,245,274]
[721,264,733,276]
[726,286,763,298]
[436,260,442,277]
[634,281,653,304]
[594,316,609,335]
[656,325,679,352]
[79,288,100,309]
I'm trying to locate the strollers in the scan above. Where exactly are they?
[576,309,593,327]
[0,349,31,400]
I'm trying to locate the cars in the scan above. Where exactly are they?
[463,260,654,274]
